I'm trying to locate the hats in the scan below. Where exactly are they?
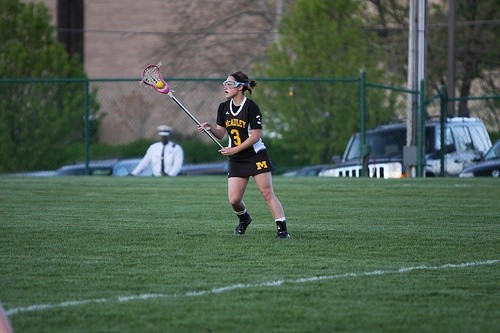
[157,124,172,136]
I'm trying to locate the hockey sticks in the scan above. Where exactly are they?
[141,65,224,149]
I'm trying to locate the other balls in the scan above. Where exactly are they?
[156,81,163,88]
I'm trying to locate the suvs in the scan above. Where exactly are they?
[317,116,493,179]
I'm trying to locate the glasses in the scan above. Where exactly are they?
[223,80,244,89]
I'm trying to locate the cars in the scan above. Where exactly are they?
[56,159,328,177]
[458,139,500,179]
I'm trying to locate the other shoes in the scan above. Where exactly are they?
[235,216,252,235]
[276,231,291,239]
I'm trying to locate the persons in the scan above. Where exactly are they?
[197,71,290,239]
[128,126,184,177]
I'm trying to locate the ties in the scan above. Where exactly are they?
[161,145,164,173]
